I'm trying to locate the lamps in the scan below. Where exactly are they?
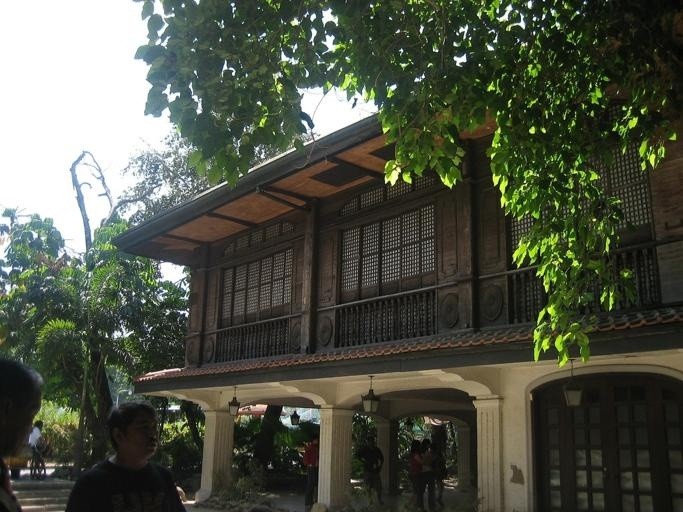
[289,398,301,426]
[561,357,584,408]
[359,375,382,414]
[226,385,240,416]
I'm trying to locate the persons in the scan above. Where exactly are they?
[410,441,428,511]
[418,438,437,511]
[301,433,319,512]
[357,435,387,506]
[0,358,45,511]
[27,418,44,477]
[433,442,445,508]
[64,399,185,512]
